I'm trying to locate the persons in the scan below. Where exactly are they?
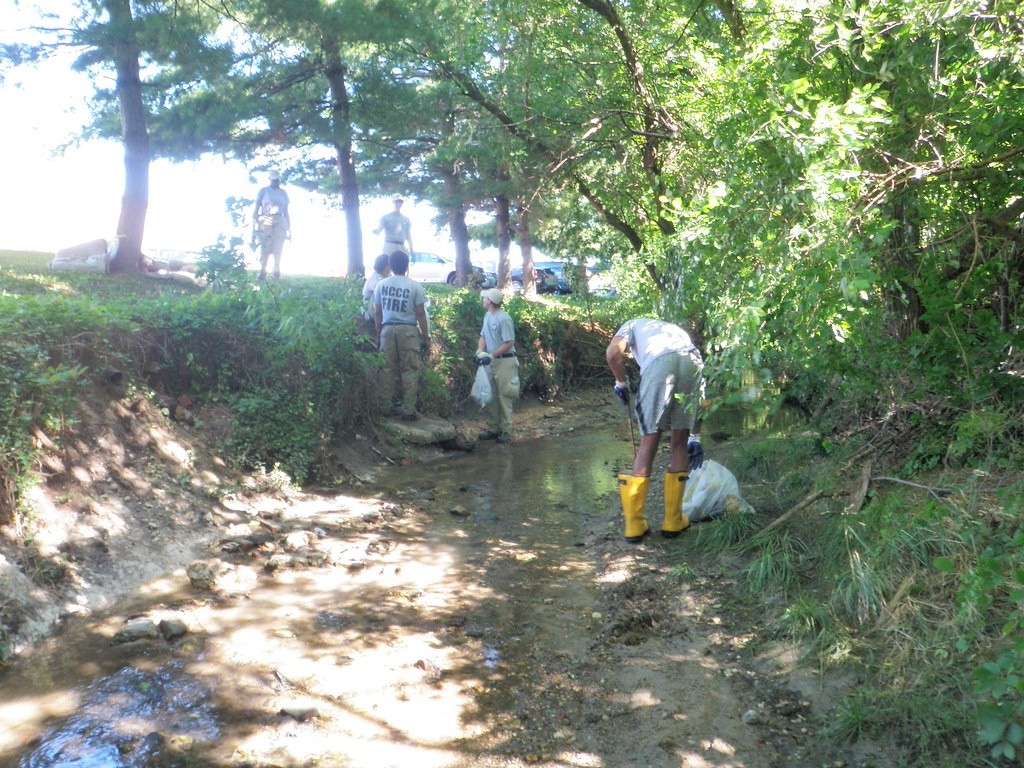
[250,168,292,286]
[358,193,418,323]
[604,315,705,543]
[471,287,520,438]
[373,249,432,419]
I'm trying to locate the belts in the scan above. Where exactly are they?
[383,322,416,325]
[386,240,404,244]
[496,353,516,358]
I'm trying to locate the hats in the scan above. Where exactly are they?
[391,194,405,202]
[269,170,280,180]
[480,288,503,306]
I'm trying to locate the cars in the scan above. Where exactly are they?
[390,251,498,289]
[510,261,618,296]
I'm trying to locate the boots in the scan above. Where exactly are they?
[661,470,690,537]
[618,473,650,541]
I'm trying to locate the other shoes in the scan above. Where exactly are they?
[497,431,509,442]
[402,412,421,420]
[480,432,497,440]
[384,409,399,415]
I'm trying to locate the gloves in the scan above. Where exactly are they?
[687,436,703,469]
[614,374,631,405]
[474,351,494,365]
